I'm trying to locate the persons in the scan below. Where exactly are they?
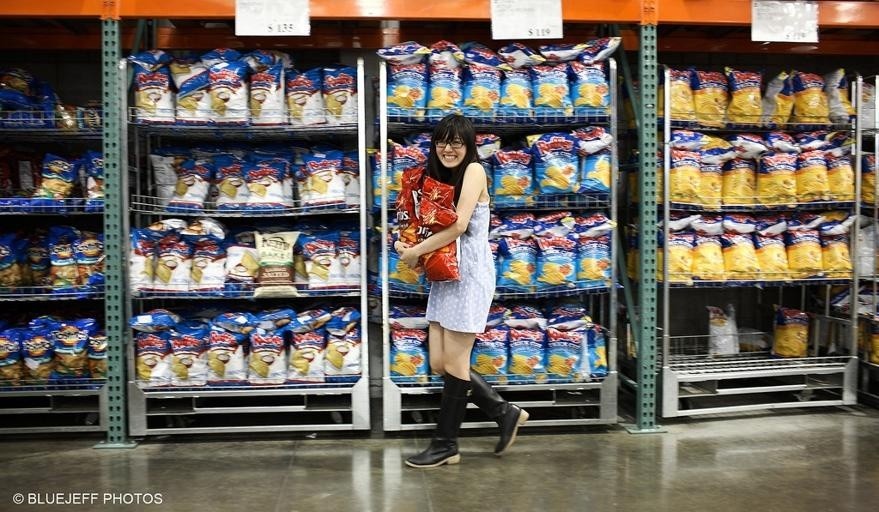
[393,114,529,469]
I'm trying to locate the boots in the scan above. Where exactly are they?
[467,366,529,456]
[404,371,473,468]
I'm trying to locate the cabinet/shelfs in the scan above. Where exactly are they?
[660,66,860,419]
[0,107,108,435]
[378,58,619,433]
[860,76,879,411]
[118,56,372,437]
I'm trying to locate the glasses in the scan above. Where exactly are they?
[435,140,465,149]
